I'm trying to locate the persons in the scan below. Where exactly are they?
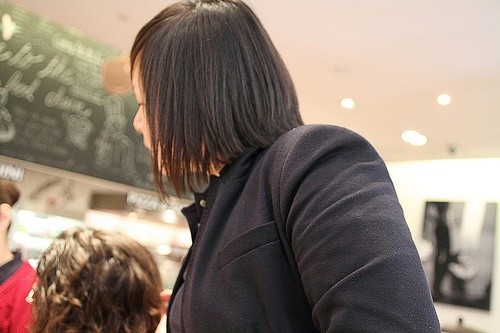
[25,227,163,333]
[129,1,442,333]
[0,181,39,333]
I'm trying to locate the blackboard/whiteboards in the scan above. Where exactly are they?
[0,1,200,202]
